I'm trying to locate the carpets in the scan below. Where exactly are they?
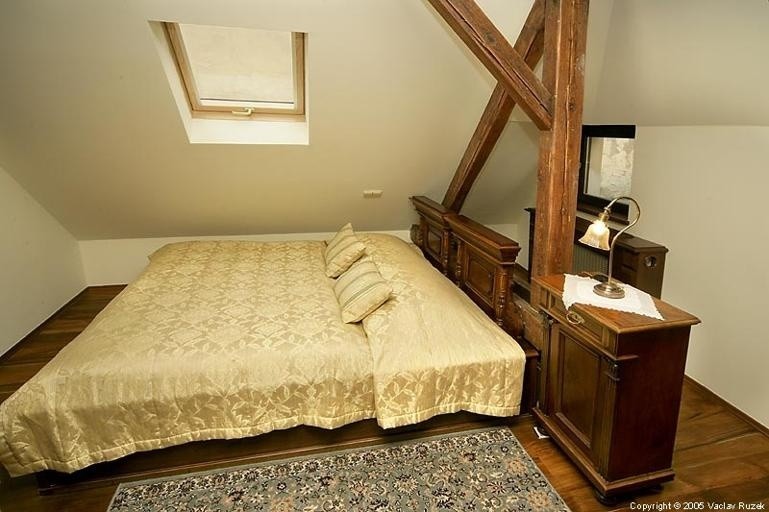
[105,424,575,512]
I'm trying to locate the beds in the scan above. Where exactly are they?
[0,232,525,494]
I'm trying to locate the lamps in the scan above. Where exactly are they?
[580,195,642,301]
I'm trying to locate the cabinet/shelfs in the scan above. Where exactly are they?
[525,203,667,300]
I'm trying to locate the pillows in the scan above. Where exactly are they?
[322,223,391,326]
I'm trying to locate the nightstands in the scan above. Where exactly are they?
[529,271,700,508]
